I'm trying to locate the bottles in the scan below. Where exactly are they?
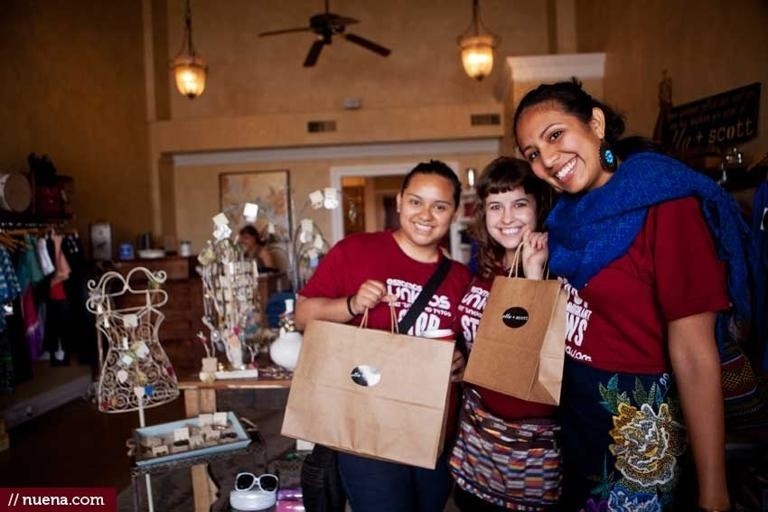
[179,240,191,257]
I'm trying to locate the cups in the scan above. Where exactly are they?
[118,245,132,260]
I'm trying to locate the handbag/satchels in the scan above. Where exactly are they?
[462,242,567,406]
[280,303,456,470]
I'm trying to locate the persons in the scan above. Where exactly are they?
[291,159,476,510]
[508,75,766,510]
[447,157,566,511]
[236,223,274,274]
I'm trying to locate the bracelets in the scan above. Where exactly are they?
[342,293,358,318]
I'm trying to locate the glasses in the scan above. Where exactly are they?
[235,472,279,492]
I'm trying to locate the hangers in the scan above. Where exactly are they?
[0,228,25,252]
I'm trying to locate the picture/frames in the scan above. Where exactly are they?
[218,169,293,242]
[88,219,114,264]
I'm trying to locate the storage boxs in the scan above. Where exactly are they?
[134,411,251,473]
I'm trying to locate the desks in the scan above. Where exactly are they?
[178,374,293,512]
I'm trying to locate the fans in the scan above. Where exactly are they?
[260,0,392,67]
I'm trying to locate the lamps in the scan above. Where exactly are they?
[457,0,502,82]
[167,0,207,100]
